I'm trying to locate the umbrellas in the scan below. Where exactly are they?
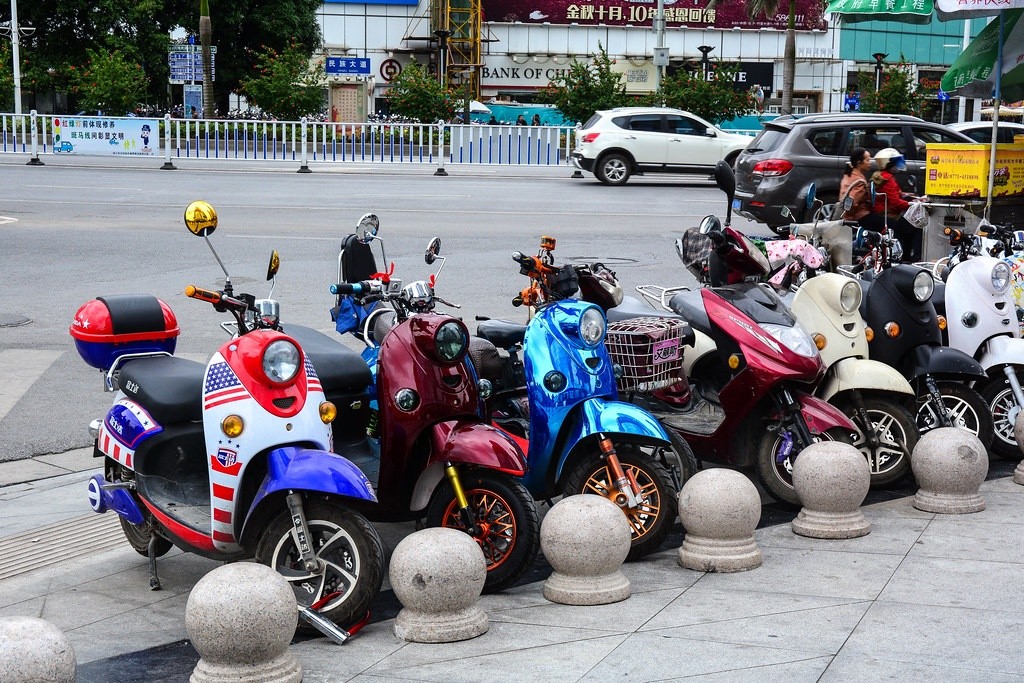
[938,8,1024,103]
[823,0,1024,222]
[454,99,491,114]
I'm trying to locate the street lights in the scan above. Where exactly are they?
[696,45,716,83]
[434,29,456,85]
[871,53,889,110]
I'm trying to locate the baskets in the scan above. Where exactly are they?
[603,316,688,393]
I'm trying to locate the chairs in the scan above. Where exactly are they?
[890,135,907,156]
[815,137,832,155]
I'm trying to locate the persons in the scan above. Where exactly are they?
[838,147,923,264]
[451,112,464,125]
[191,106,197,119]
[489,114,542,126]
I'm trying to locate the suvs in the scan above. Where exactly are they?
[572,106,756,187]
[731,110,979,236]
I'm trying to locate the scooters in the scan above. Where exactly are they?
[66,158,1024,650]
[366,110,512,134]
[135,103,330,122]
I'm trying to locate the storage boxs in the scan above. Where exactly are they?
[69,295,180,369]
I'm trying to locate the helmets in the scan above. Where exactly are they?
[873,147,904,169]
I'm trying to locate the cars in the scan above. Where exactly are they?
[922,120,1024,145]
